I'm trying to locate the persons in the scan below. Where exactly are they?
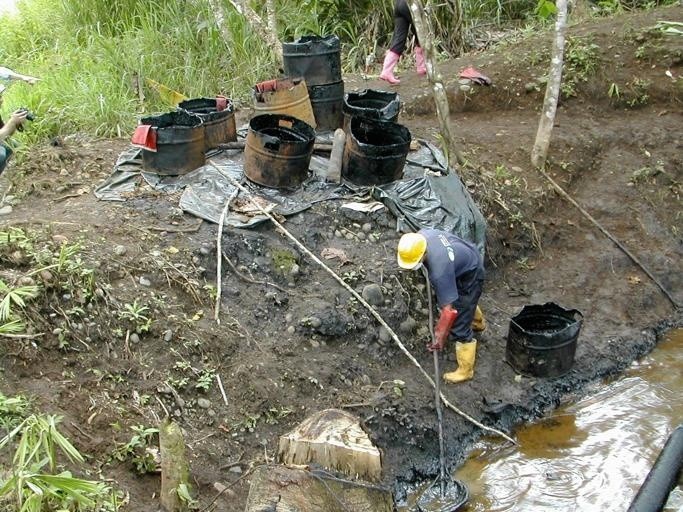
[397,228,487,385]
[378,1,428,84]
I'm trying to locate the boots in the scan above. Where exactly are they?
[471,305,485,331]
[443,338,476,383]
[416,47,426,73]
[380,51,400,85]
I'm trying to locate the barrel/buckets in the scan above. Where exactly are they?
[178,97,237,152]
[505,301,585,379]
[282,34,344,132]
[252,75,318,131]
[341,87,402,133]
[138,111,204,176]
[341,114,412,186]
[243,113,315,188]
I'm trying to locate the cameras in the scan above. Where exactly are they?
[16,106,35,121]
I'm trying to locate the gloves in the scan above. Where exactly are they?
[461,67,491,86]
[427,308,457,350]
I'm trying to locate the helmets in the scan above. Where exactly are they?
[396,232,427,269]
